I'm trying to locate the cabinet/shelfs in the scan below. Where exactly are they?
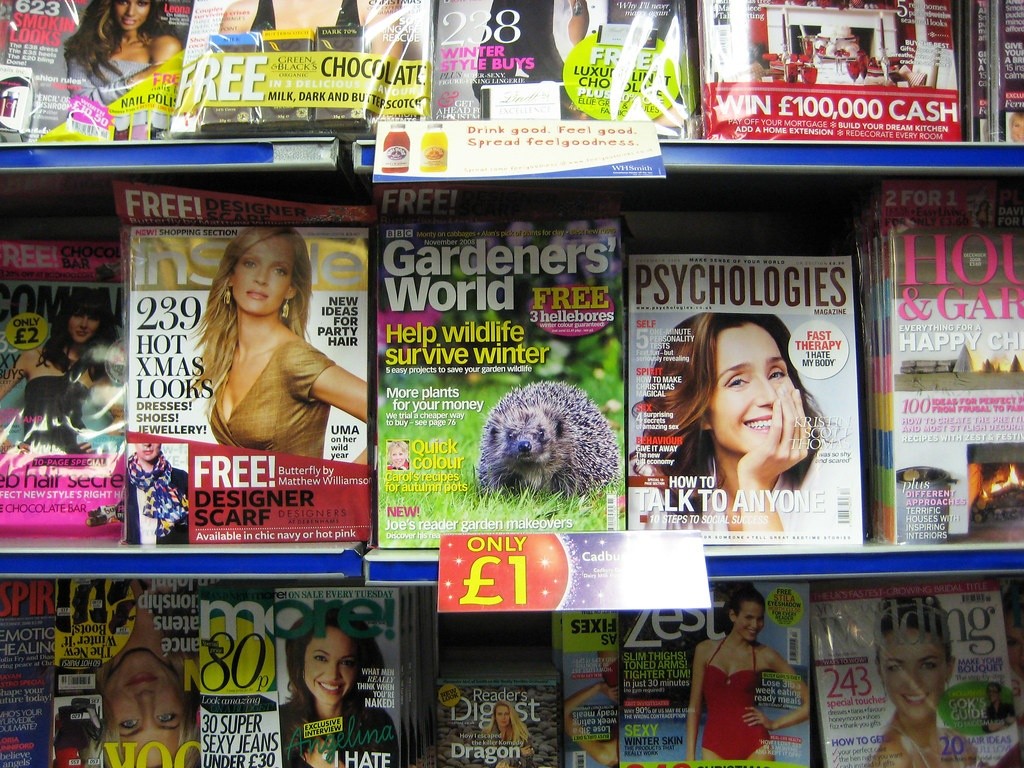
[350,137,1024,589]
[0,136,365,581]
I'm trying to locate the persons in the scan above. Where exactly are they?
[387,441,410,471]
[867,592,988,768]
[564,659,620,768]
[482,701,534,768]
[0,288,127,454]
[94,592,200,768]
[127,441,189,544]
[686,587,809,762]
[188,223,367,467]
[278,605,398,768]
[640,311,825,531]
[980,681,1016,734]
[219,0,404,60]
[64,0,182,141]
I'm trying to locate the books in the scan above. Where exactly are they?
[0,0,1024,768]
